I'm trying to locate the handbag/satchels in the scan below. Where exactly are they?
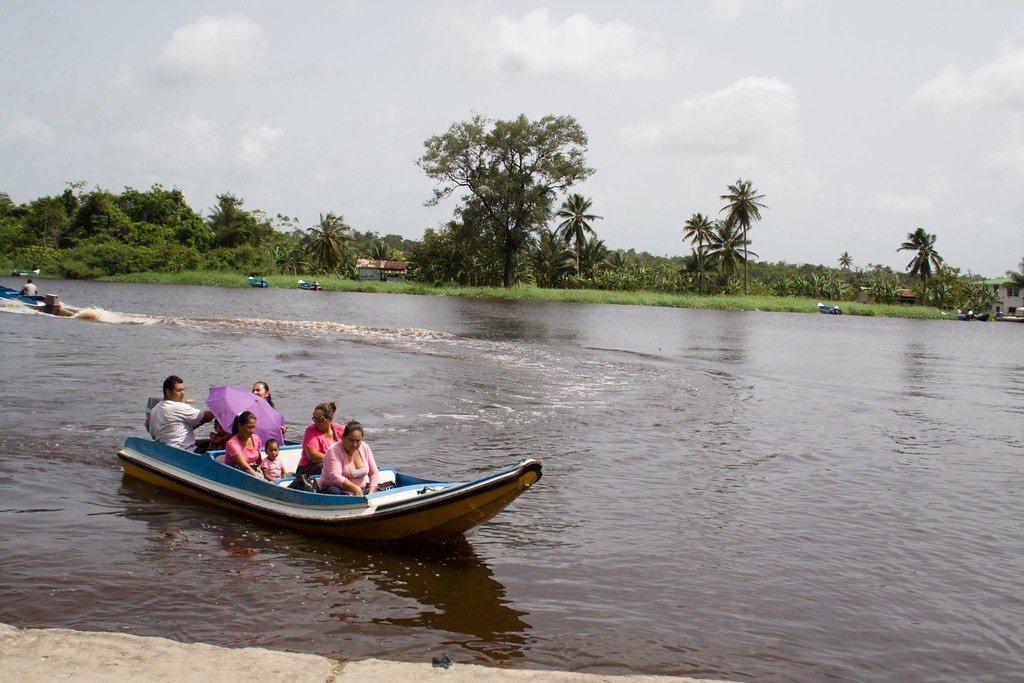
[289,474,318,493]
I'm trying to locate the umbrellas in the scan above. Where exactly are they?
[205,385,286,447]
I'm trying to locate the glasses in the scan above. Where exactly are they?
[312,417,327,423]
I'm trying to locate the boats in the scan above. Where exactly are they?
[0,284,62,315]
[248,276,270,288]
[116,396,546,546]
[942,309,991,321]
[818,302,842,316]
[994,311,1024,322]
[11,269,41,278]
[297,279,323,292]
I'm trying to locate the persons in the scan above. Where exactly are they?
[149,376,215,454]
[208,381,285,450]
[22,279,38,295]
[319,419,379,496]
[260,439,286,481]
[225,411,262,476]
[296,402,346,475]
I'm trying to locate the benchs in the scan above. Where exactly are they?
[5,291,24,295]
[275,468,398,489]
[206,444,304,474]
